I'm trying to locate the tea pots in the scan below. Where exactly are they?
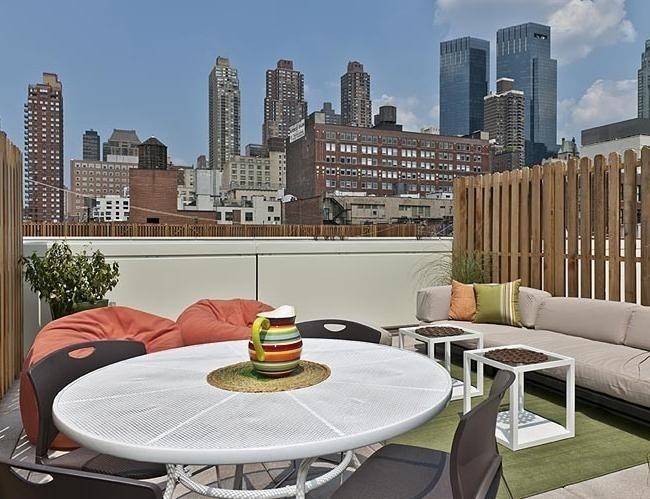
[248,304,306,378]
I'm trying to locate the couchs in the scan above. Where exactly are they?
[416,285,650,428]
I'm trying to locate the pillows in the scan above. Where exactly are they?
[518,286,552,329]
[535,297,636,345]
[417,285,452,322]
[472,278,523,326]
[449,278,476,321]
[625,304,650,352]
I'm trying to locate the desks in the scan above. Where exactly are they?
[398,325,484,401]
[462,345,576,453]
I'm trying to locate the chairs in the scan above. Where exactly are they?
[20,306,184,450]
[0,457,163,499]
[26,341,189,479]
[295,319,381,344]
[175,298,276,346]
[330,370,516,499]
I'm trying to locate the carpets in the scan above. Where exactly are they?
[379,359,650,499]
